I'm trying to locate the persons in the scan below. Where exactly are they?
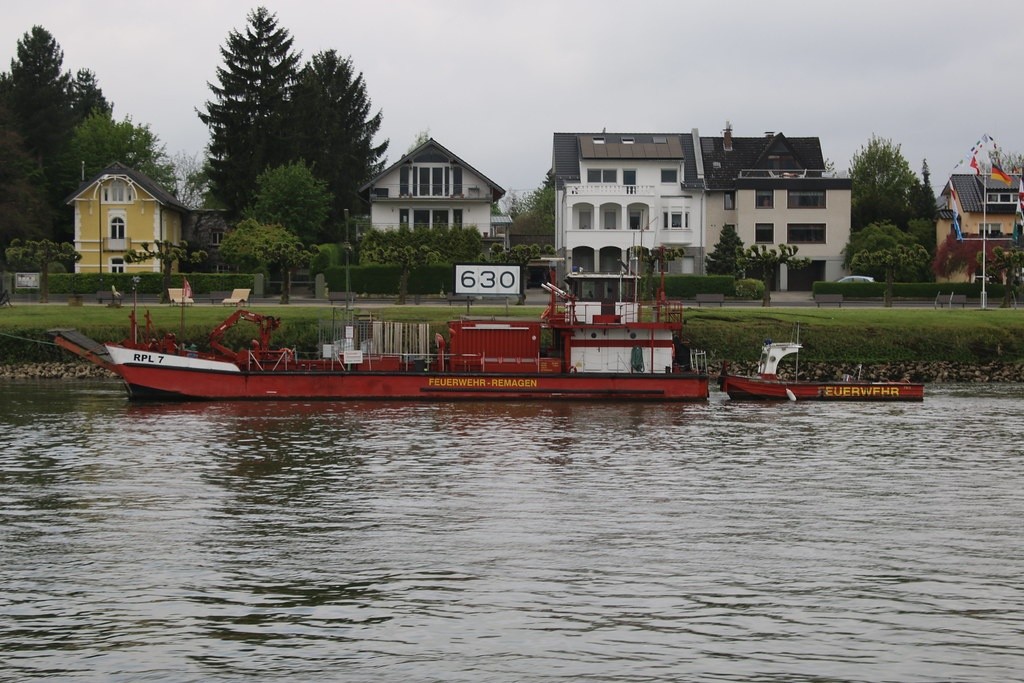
[148,337,163,348]
[177,340,198,357]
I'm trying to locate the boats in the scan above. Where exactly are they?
[717,319,926,404]
[98,202,715,401]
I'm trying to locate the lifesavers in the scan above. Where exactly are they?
[279,348,293,364]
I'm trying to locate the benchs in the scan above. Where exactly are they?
[210,291,232,304]
[222,288,252,306]
[695,294,724,307]
[814,294,844,308]
[938,295,967,308]
[168,288,195,306]
[96,291,124,305]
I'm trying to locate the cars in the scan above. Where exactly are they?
[832,275,874,283]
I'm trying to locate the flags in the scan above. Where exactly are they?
[948,179,964,243]
[970,156,981,176]
[1016,177,1024,226]
[991,161,1013,185]
[184,279,193,297]
